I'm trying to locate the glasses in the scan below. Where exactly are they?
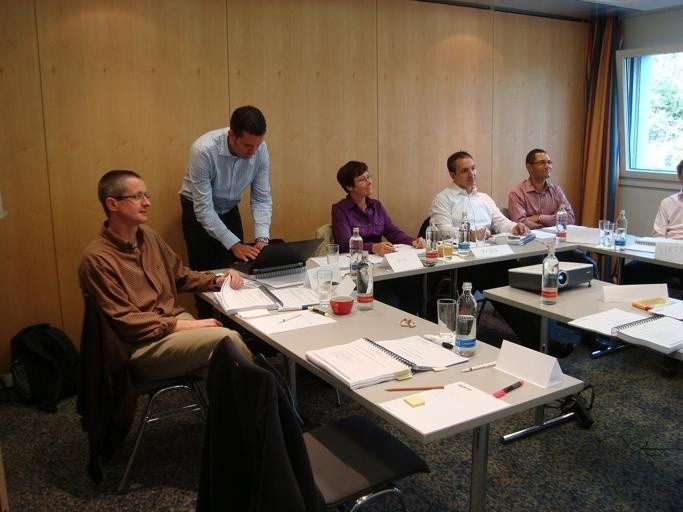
[528,160,552,165]
[353,174,370,183]
[110,192,151,201]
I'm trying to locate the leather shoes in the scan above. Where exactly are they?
[298,411,321,430]
[664,356,679,377]
[548,339,575,358]
[555,334,581,345]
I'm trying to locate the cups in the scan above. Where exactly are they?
[598,219,610,249]
[603,221,615,250]
[317,270,332,309]
[437,298,457,341]
[326,244,339,270]
[442,231,453,259]
[330,296,354,315]
[475,225,486,247]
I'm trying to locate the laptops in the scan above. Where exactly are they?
[225,235,325,276]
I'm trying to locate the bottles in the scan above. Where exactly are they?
[349,228,363,276]
[556,204,567,242]
[459,212,471,255]
[455,282,476,357]
[542,246,559,305]
[357,252,373,310]
[426,221,439,264]
[615,209,628,250]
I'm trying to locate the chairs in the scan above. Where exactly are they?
[81,294,216,494]
[311,226,336,261]
[200,336,430,511]
[419,219,503,324]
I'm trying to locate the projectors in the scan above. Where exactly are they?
[508,260,594,294]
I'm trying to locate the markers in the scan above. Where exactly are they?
[279,305,307,311]
[492,379,523,398]
[424,334,453,348]
[632,301,652,311]
[307,306,329,317]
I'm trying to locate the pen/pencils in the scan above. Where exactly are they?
[384,386,444,390]
[282,311,302,322]
[498,235,521,239]
[460,361,496,371]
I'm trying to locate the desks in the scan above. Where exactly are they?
[536,226,682,357]
[303,226,577,321]
[193,271,584,511]
[482,277,683,444]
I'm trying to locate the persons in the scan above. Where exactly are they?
[430,150,530,243]
[178,106,274,318]
[508,149,576,230]
[79,170,253,377]
[653,160,683,241]
[331,159,425,256]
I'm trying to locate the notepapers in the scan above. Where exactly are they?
[403,395,425,406]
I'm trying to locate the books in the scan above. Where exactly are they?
[485,233,536,246]
[620,237,672,253]
[310,253,383,269]
[212,274,320,316]
[306,335,470,390]
[568,307,683,356]
[527,224,565,244]
